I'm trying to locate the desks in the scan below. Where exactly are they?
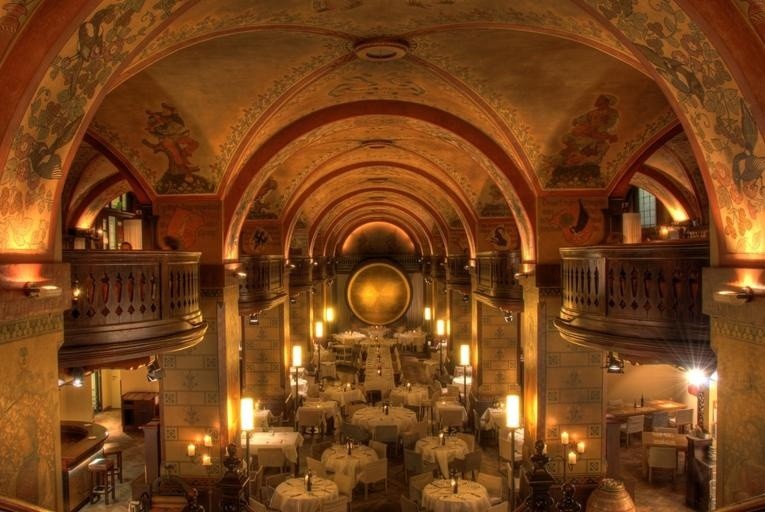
[641,431,688,478]
[607,399,688,444]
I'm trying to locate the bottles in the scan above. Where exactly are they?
[308,468,312,485]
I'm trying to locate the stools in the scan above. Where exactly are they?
[103,442,123,484]
[88,458,115,505]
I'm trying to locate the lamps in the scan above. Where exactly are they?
[291,345,301,410]
[437,320,445,377]
[506,396,519,512]
[461,344,470,407]
[316,322,323,378]
[240,397,254,505]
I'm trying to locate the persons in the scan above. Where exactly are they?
[160,236,178,250]
[119,242,131,249]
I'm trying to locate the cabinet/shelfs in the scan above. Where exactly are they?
[121,391,159,433]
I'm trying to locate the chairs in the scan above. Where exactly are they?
[646,446,678,485]
[644,411,669,435]
[619,415,646,448]
[608,399,624,406]
[669,408,694,433]
[238,327,524,512]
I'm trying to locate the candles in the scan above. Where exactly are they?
[569,452,577,465]
[203,455,210,465]
[561,432,568,444]
[189,445,195,457]
[204,434,212,447]
[577,442,585,453]
